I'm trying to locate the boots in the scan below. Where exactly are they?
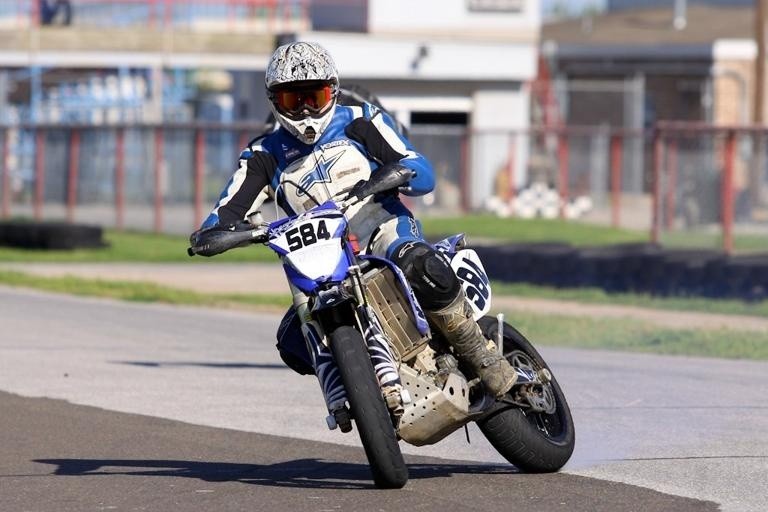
[427,286,518,397]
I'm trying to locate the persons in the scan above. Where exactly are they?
[190,40,518,397]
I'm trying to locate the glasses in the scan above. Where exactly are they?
[266,85,337,118]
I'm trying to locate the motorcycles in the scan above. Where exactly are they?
[187,165,577,489]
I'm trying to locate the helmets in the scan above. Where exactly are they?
[266,42,340,143]
